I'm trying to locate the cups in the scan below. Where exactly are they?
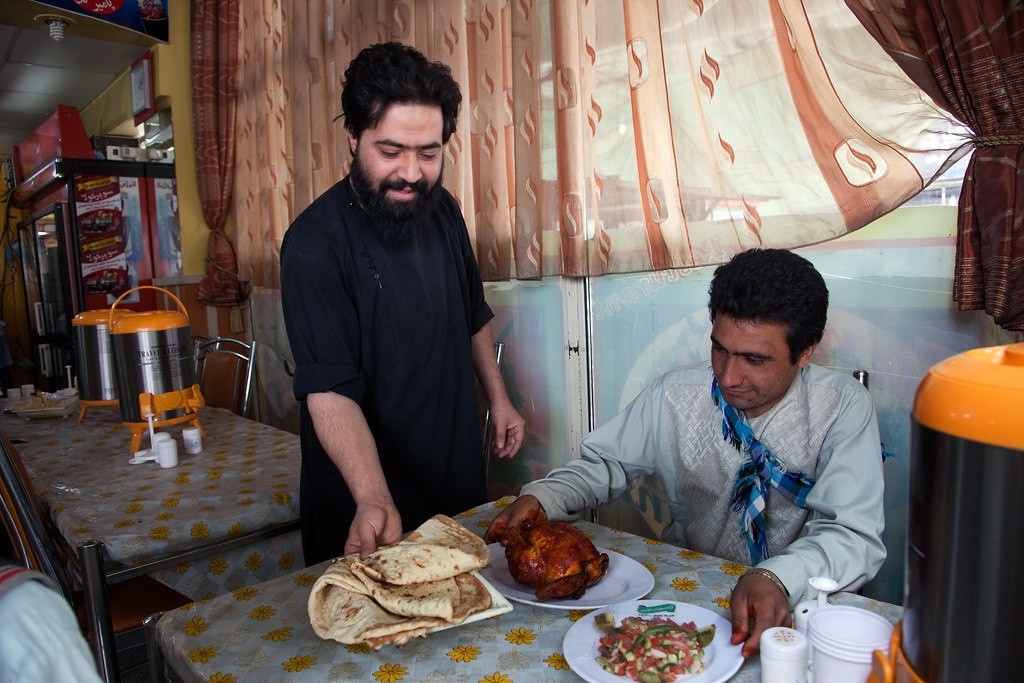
[808,604,893,683]
[7,388,21,398]
[21,384,34,400]
[150,425,204,468]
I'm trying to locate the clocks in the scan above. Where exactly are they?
[129,49,154,127]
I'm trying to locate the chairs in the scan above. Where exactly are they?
[0,429,194,678]
[190,333,256,420]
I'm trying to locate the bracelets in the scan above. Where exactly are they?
[739,568,789,604]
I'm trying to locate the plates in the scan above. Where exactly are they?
[478,542,655,609]
[11,396,80,420]
[329,549,514,632]
[563,599,745,683]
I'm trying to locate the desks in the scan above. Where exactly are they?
[143,495,906,683]
[0,395,305,682]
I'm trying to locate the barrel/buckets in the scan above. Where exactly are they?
[72,308,137,425]
[863,342,1024,683]
[109,285,208,455]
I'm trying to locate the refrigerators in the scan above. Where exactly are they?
[11,105,180,392]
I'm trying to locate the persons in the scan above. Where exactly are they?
[280,42,526,568]
[483,248,887,657]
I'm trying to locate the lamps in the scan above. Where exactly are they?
[49,20,64,41]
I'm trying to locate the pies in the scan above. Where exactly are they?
[13,394,54,408]
[308,515,492,650]
